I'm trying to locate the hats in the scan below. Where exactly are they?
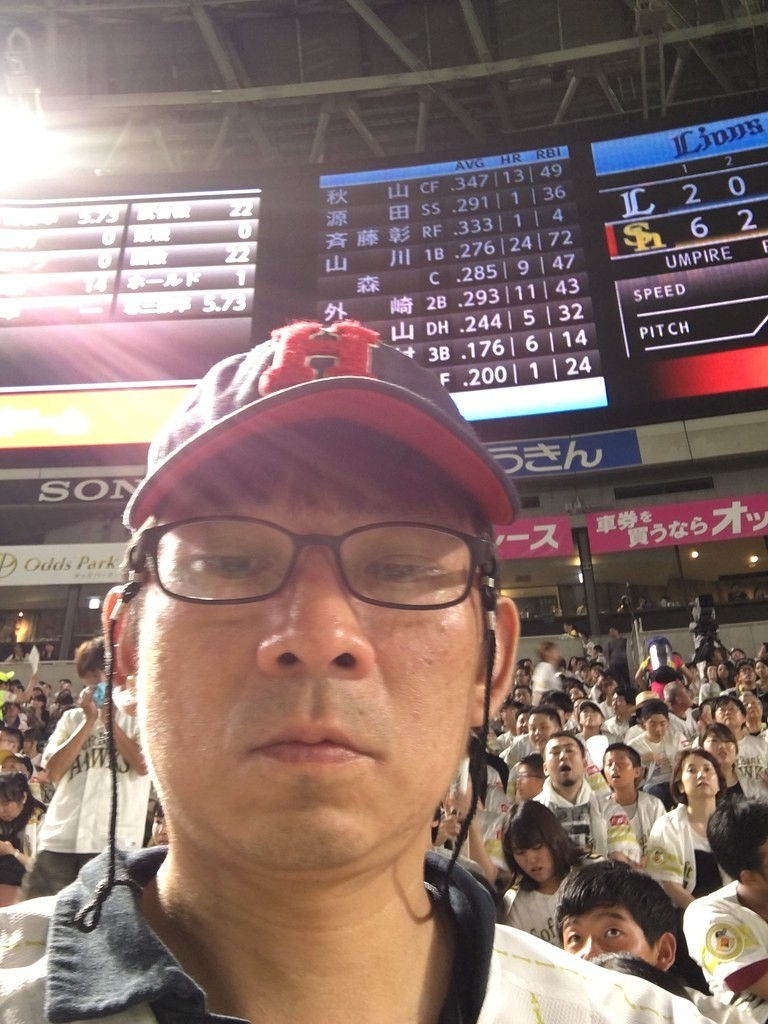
[0,749,33,780]
[628,691,659,713]
[579,700,607,722]
[0,671,15,685]
[123,320,522,526]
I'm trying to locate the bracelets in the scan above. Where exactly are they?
[15,849,19,858]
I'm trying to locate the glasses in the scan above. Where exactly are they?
[128,516,498,610]
[516,772,545,782]
[716,706,739,714]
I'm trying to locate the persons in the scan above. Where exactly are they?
[86,321,723,1024]
[0,583,768,1024]
